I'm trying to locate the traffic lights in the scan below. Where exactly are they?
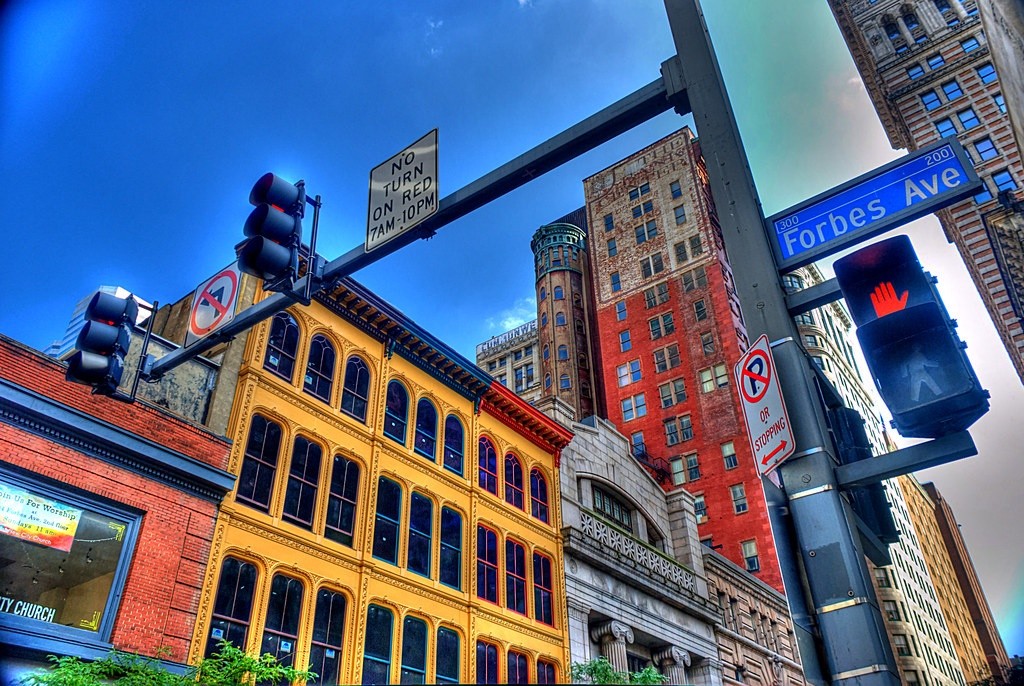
[65,290,139,396]
[237,172,306,293]
[832,234,992,440]
